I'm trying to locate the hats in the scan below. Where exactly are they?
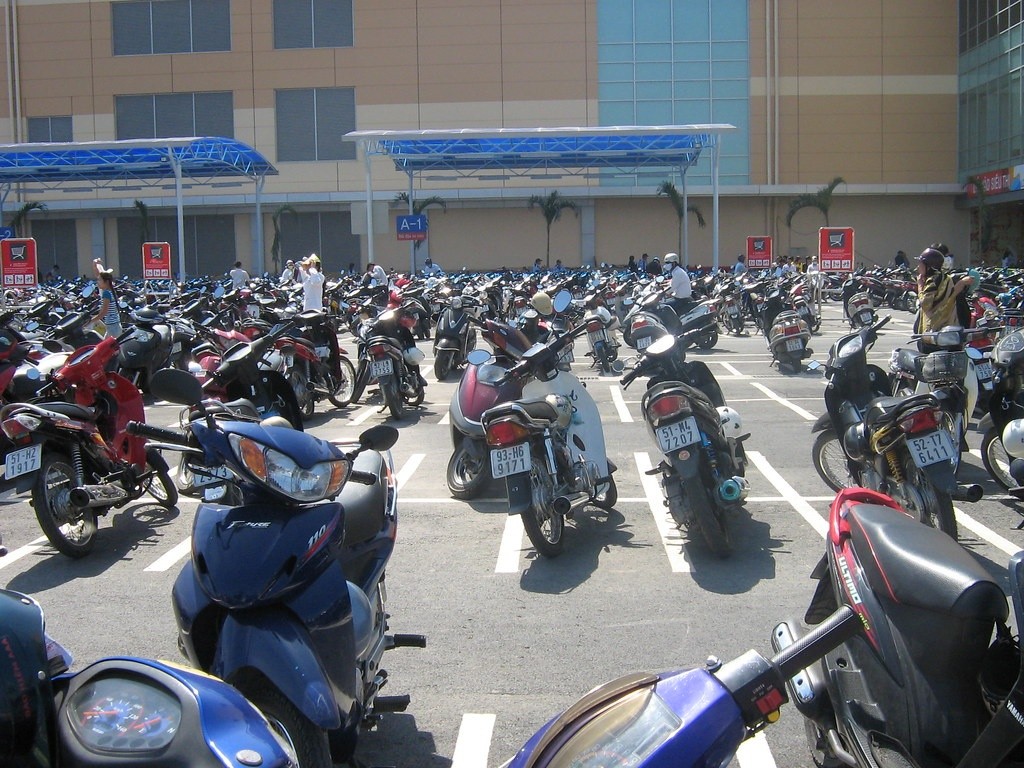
[931,242,952,258]
[536,259,542,263]
[812,256,817,260]
[286,260,293,264]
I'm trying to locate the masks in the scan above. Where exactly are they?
[664,264,672,270]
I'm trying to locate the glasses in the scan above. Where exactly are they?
[665,262,673,264]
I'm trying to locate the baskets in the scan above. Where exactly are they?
[919,351,967,383]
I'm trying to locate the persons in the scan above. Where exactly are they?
[1002,250,1024,269]
[424,257,443,277]
[533,258,543,272]
[280,257,308,295]
[553,259,567,272]
[771,255,812,273]
[734,254,750,276]
[348,263,356,276]
[45,265,60,283]
[299,254,325,314]
[629,254,663,276]
[895,250,910,269]
[367,263,388,287]
[656,253,692,317]
[230,262,252,291]
[916,243,976,353]
[93,258,123,340]
[806,256,826,303]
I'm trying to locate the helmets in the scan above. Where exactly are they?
[716,407,742,438]
[0,589,56,768]
[542,393,572,428]
[914,248,944,270]
[654,257,659,260]
[751,293,758,300]
[966,266,980,295]
[844,420,869,462]
[403,348,424,365]
[716,295,725,304]
[664,253,679,264]
[531,292,551,315]
[1003,419,1024,459]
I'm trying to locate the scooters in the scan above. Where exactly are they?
[0,255,1024,768]
